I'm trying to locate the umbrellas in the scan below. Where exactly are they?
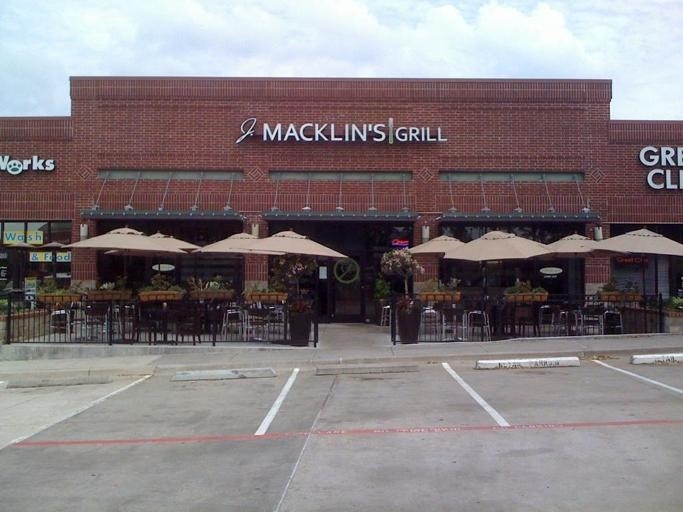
[4,242,35,249]
[546,231,598,334]
[441,230,554,332]
[406,235,465,286]
[227,229,350,338]
[589,226,682,332]
[37,241,65,249]
[190,231,288,326]
[59,224,189,289]
[102,230,202,290]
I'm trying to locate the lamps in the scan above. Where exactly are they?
[594,226,603,241]
[422,226,429,244]
[80,224,88,242]
[251,223,259,237]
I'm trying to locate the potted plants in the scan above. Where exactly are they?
[418,277,462,303]
[504,280,549,303]
[380,246,426,344]
[598,277,642,301]
[33,275,289,304]
[271,254,319,346]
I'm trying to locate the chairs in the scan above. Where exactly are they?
[418,295,624,342]
[50,301,284,342]
